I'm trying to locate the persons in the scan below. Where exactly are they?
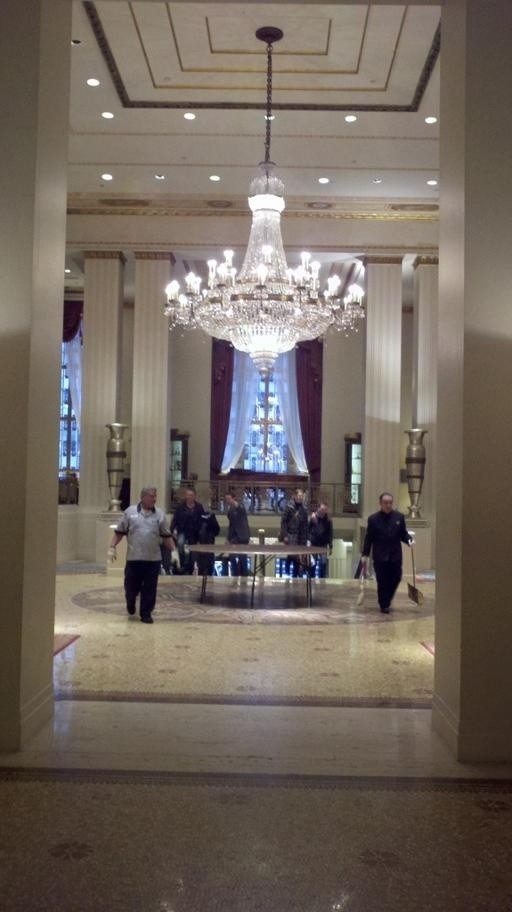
[224,491,252,575]
[278,488,309,577]
[106,485,181,624]
[360,492,415,613]
[309,501,334,577]
[170,486,204,574]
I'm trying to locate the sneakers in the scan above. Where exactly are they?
[381,606,388,613]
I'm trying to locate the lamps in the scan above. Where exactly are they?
[162,27,365,378]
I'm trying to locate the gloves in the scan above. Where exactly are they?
[108,545,116,563]
[171,549,180,568]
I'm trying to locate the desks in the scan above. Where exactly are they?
[215,473,308,511]
[186,544,327,608]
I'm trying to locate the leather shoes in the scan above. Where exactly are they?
[140,614,153,622]
[127,603,135,614]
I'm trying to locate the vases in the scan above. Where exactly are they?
[105,423,128,512]
[403,428,429,519]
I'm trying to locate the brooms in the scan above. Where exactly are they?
[357,563,367,607]
